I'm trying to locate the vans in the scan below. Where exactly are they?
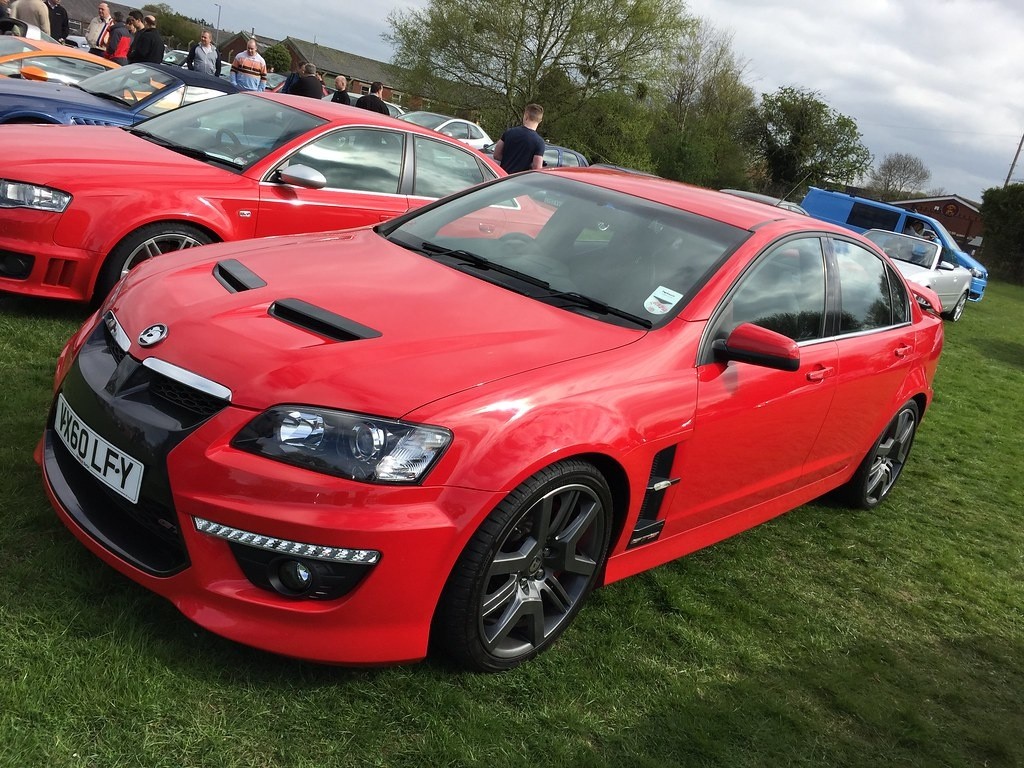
[799,186,988,302]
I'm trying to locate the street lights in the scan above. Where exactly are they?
[214,4,221,47]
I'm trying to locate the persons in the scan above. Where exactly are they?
[229,38,268,91]
[906,219,939,244]
[283,61,391,117]
[186,30,222,77]
[84,2,165,67]
[0,0,70,43]
[492,103,547,174]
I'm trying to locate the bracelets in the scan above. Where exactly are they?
[930,235,933,238]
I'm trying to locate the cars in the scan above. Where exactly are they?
[0,62,283,150]
[160,50,405,119]
[836,228,972,322]
[718,189,811,218]
[0,35,181,111]
[453,142,589,184]
[32,168,943,673]
[534,163,662,231]
[382,111,494,160]
[0,92,559,311]
